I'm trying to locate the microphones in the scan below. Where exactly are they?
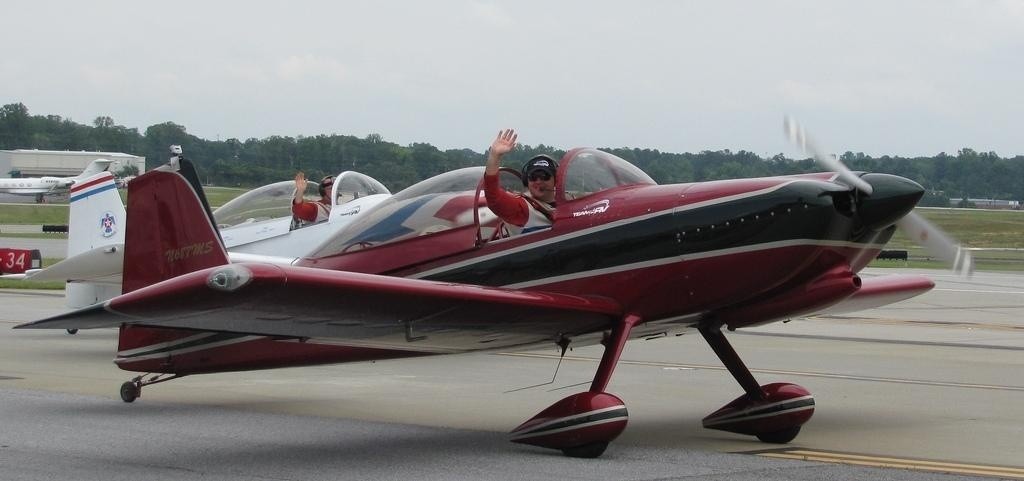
[540,186,556,192]
[324,193,332,202]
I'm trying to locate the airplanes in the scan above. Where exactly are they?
[0,158,137,204]
[13,116,975,458]
[0,157,494,308]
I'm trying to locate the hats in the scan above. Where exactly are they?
[527,157,554,176]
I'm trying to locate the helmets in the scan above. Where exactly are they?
[319,176,337,196]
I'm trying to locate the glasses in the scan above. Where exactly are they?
[528,174,550,180]
[321,176,335,186]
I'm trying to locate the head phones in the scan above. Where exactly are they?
[521,153,558,185]
[318,174,334,196]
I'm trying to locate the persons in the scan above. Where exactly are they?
[292,171,336,223]
[483,128,559,237]
